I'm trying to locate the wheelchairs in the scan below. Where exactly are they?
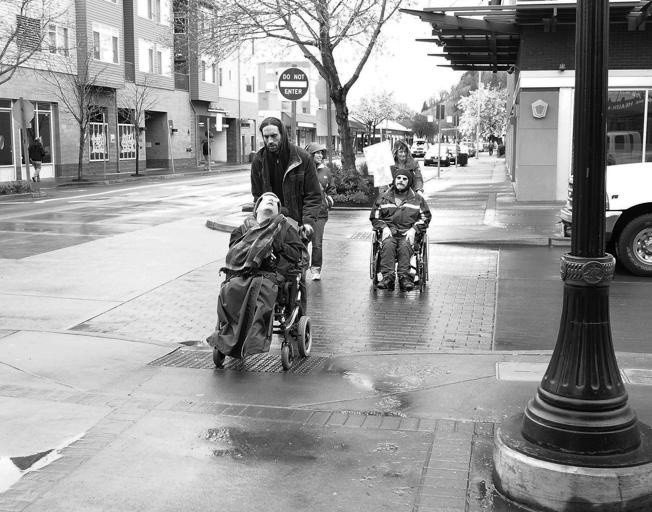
[364,218,430,294]
[210,222,316,369]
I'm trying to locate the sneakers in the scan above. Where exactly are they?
[310,265,322,281]
[37,179,40,182]
[32,176,36,182]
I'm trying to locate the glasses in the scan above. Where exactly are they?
[262,195,279,202]
[395,177,408,180]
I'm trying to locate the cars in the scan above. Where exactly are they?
[409,139,491,166]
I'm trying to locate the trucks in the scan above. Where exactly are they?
[606,130,650,164]
[543,161,651,276]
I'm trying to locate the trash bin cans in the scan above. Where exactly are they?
[250,152,256,162]
[459,153,468,166]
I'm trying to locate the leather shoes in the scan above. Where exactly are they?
[377,277,393,289]
[399,275,415,289]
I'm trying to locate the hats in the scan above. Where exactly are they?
[253,192,281,218]
[393,169,411,192]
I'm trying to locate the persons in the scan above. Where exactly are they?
[28,137,44,182]
[203,140,211,170]
[304,141,336,281]
[390,139,428,202]
[206,191,303,358]
[368,169,432,290]
[250,116,322,335]
[488,140,494,156]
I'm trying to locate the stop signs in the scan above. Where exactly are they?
[278,69,309,101]
